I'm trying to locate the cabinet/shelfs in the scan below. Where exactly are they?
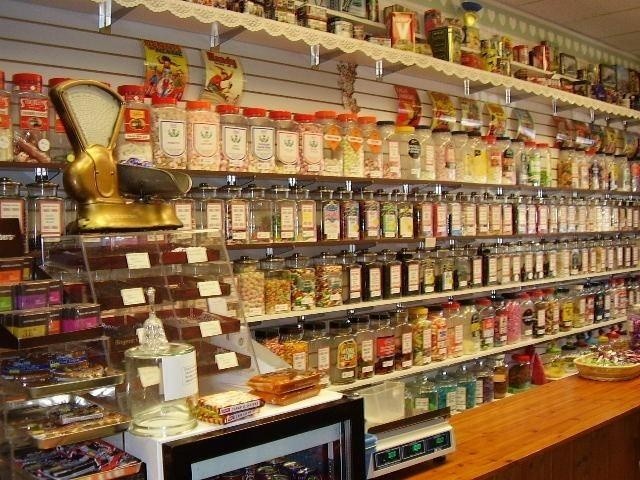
[394,371,638,480]
[2,0,640,479]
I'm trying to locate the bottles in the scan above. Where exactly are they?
[573,285,586,326]
[269,111,300,174]
[259,255,292,314]
[196,183,223,235]
[525,141,539,186]
[123,343,199,436]
[321,190,341,241]
[307,323,328,376]
[382,249,402,297]
[538,144,551,188]
[513,194,640,235]
[558,286,574,330]
[226,185,251,243]
[447,302,464,357]
[357,117,383,177]
[29,182,64,251]
[587,276,640,322]
[472,361,494,403]
[533,293,544,337]
[341,192,360,241]
[177,190,195,231]
[273,185,298,242]
[496,300,507,346]
[215,103,248,170]
[373,314,395,374]
[142,287,168,351]
[287,253,315,311]
[354,316,374,380]
[377,120,400,179]
[242,109,276,174]
[505,295,520,343]
[561,335,579,372]
[297,188,317,241]
[245,184,273,243]
[312,252,341,306]
[315,111,342,176]
[150,98,187,170]
[395,127,420,180]
[481,135,502,183]
[431,128,455,181]
[519,294,534,335]
[115,87,152,165]
[329,319,357,384]
[440,239,544,290]
[186,100,221,171]
[574,324,632,356]
[232,256,264,315]
[543,289,559,334]
[542,343,563,377]
[413,307,432,365]
[402,248,419,295]
[47,78,83,164]
[511,139,528,185]
[405,378,437,414]
[0,71,13,161]
[293,113,323,176]
[451,131,472,183]
[485,355,509,398]
[357,250,381,300]
[462,302,483,355]
[508,354,532,393]
[0,177,27,257]
[393,310,413,369]
[545,235,640,280]
[254,325,312,370]
[479,299,496,349]
[421,251,435,293]
[557,145,640,193]
[11,73,51,164]
[338,251,362,304]
[414,125,434,180]
[456,364,477,411]
[494,136,515,183]
[362,191,380,239]
[428,307,449,361]
[466,132,486,184]
[378,190,514,239]
[434,371,458,413]
[338,114,363,177]
